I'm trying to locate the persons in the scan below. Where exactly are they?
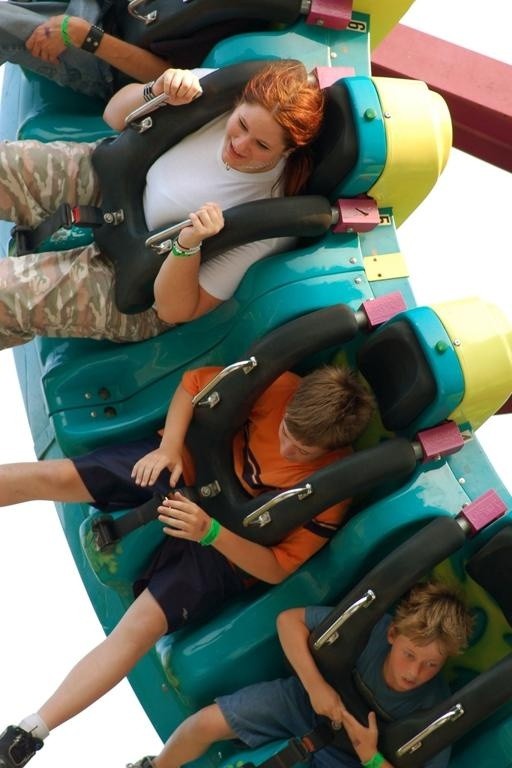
[126,583,475,768]
[0,0,305,83]
[0,364,376,768]
[0,59,325,350]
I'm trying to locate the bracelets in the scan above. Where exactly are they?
[200,518,221,546]
[81,24,104,54]
[361,751,384,768]
[172,235,202,257]
[143,80,155,102]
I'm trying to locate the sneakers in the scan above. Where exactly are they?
[125,754,156,768]
[0,724,46,768]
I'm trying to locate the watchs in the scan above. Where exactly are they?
[61,16,73,49]
